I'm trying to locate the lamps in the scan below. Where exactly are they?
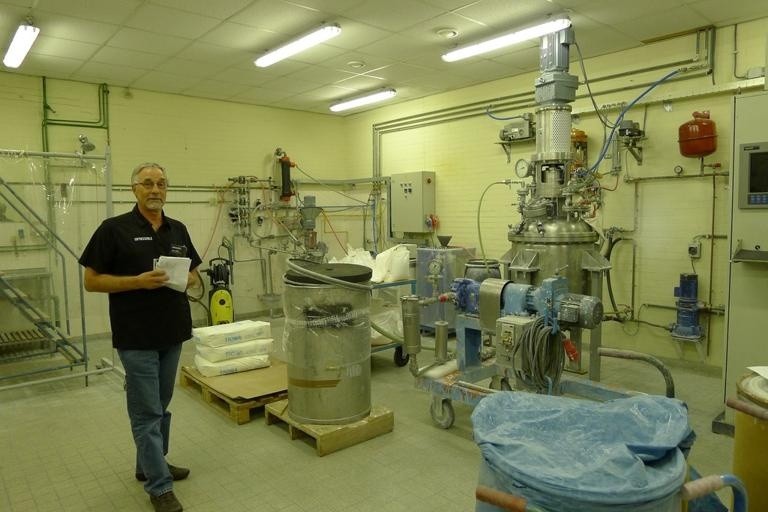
[2,13,41,69]
[79,134,95,155]
[252,21,341,68]
[124,87,132,98]
[329,86,396,113]
[440,13,571,62]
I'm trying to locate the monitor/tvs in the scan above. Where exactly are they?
[738,142,768,209]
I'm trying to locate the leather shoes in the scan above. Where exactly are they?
[150,491,183,512]
[136,463,190,481]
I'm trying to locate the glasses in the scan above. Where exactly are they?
[131,180,166,189]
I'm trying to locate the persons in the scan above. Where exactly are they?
[77,160,205,511]
